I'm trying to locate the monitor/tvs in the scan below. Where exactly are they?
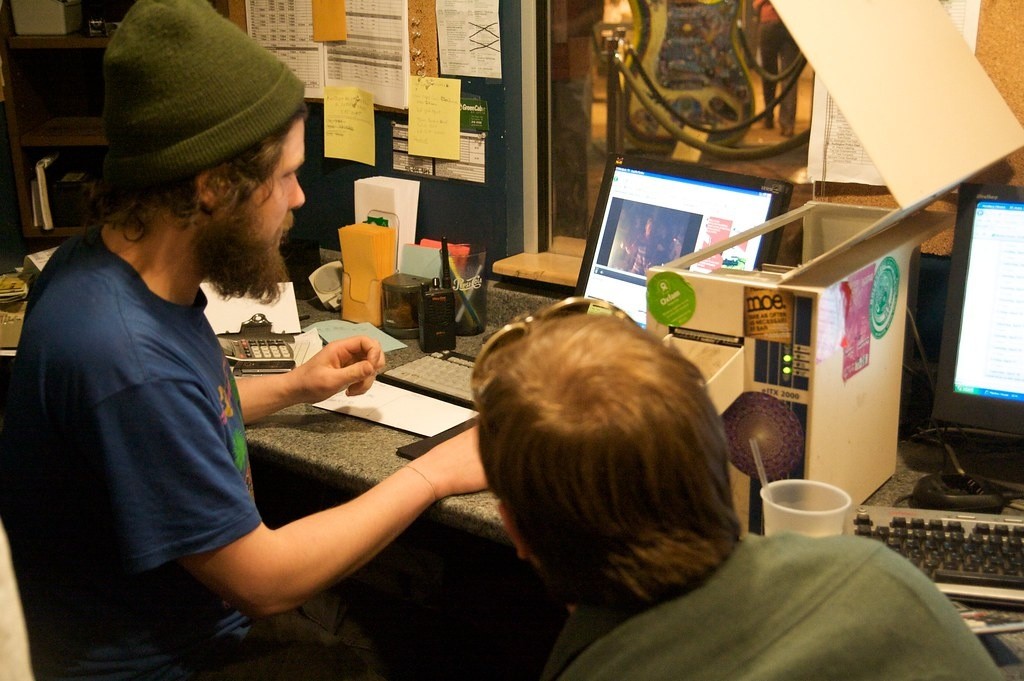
[574,151,796,331]
[930,180,1024,500]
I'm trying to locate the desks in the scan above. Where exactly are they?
[220,282,516,547]
[855,436,1024,681]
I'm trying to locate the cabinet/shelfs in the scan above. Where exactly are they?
[0,0,248,236]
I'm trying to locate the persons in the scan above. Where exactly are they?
[610,209,683,277]
[3,0,490,680]
[474,312,1010,681]
[751,0,804,137]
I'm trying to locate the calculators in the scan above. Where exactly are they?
[216,337,295,361]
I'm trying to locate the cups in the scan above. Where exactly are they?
[438,244,488,336]
[760,478,851,537]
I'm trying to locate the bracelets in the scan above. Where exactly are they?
[404,463,439,501]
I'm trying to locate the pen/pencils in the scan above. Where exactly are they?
[439,248,487,330]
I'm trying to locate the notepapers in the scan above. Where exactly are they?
[400,239,470,287]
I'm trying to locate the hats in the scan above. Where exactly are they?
[101,0,305,192]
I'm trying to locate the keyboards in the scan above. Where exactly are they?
[851,506,1024,612]
[376,347,478,412]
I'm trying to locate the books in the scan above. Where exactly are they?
[28,153,70,230]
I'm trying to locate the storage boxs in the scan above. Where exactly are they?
[11,0,81,36]
